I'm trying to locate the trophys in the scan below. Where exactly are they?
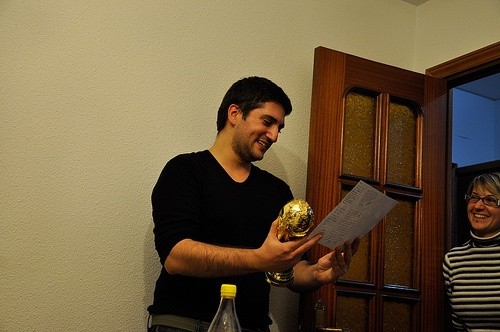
[265,199,314,287]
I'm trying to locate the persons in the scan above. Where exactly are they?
[441,173,500,332]
[148,75,363,332]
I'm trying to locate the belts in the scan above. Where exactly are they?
[150,315,211,332]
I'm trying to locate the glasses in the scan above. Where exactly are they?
[465,194,500,207]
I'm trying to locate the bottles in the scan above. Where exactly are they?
[208,284,241,332]
